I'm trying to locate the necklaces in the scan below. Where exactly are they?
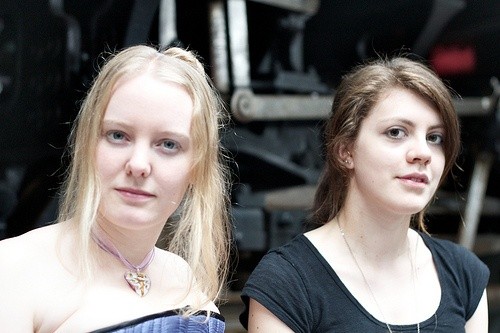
[90,226,157,297]
[336,213,421,333]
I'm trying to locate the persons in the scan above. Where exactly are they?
[238,57,491,333]
[0,43,237,333]
